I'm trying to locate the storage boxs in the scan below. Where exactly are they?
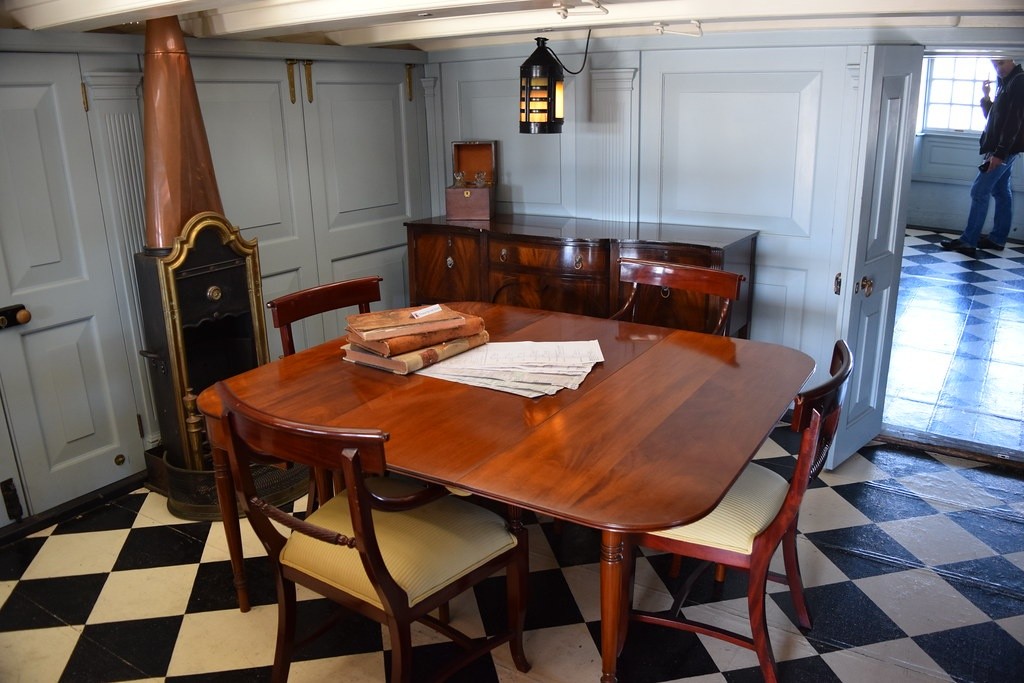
[445,139,499,221]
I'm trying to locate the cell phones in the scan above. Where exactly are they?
[978,162,990,174]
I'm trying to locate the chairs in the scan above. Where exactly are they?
[265,276,384,509]
[608,257,746,337]
[214,381,533,683]
[617,338,854,683]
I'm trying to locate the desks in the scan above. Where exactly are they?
[196,302,816,683]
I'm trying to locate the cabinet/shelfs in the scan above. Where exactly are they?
[403,213,761,341]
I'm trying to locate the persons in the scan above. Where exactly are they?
[940,59,1024,254]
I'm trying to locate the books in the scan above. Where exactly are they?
[340,304,490,375]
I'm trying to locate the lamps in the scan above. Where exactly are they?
[519,30,592,134]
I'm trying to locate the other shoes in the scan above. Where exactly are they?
[941,239,976,253]
[976,237,1004,251]
